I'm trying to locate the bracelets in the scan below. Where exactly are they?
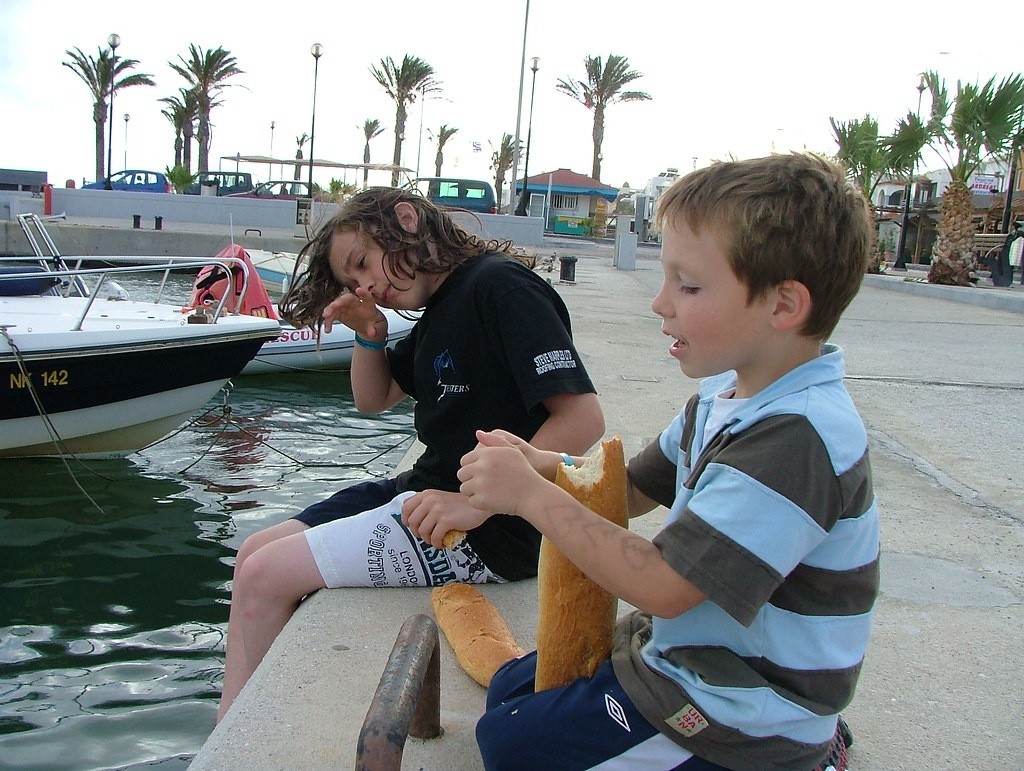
[354,331,389,351]
[558,452,574,466]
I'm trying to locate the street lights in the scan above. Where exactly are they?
[891,74,928,269]
[268,121,276,181]
[104,34,121,190]
[514,57,541,217]
[123,113,130,172]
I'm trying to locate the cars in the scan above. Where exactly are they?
[184,171,252,196]
[400,177,498,215]
[223,180,329,202]
[80,169,171,194]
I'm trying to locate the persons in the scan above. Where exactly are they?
[457,146,884,771]
[216,186,605,725]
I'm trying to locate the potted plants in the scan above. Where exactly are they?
[882,227,898,262]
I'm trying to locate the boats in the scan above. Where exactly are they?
[0,254,284,459]
[238,303,426,374]
[241,247,310,294]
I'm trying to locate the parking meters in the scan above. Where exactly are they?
[308,42,325,199]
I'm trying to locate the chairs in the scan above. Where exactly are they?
[136,176,143,185]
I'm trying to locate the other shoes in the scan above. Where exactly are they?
[813,714,854,771]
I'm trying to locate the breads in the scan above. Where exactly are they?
[430,581,525,688]
[442,529,467,550]
[534,434,630,693]
